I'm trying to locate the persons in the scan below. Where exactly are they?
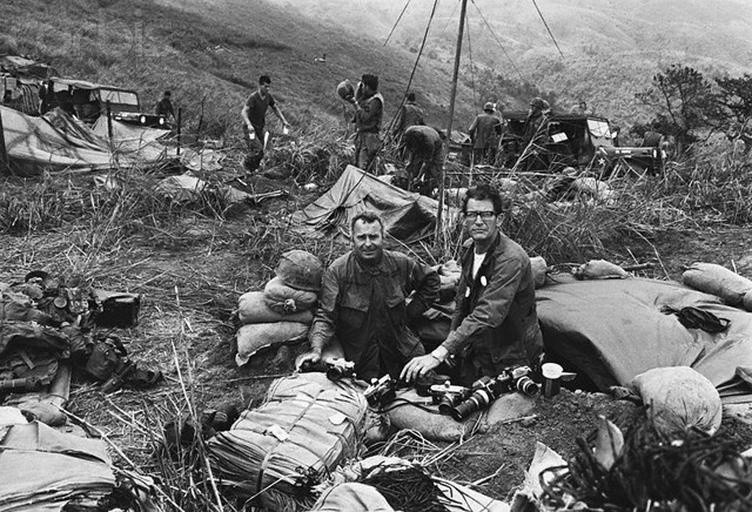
[394,125,445,199]
[239,74,292,177]
[642,122,666,149]
[467,96,553,171]
[397,184,547,388]
[336,73,385,175]
[155,90,177,124]
[392,91,426,145]
[293,211,442,383]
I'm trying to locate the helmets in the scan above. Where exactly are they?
[543,100,550,108]
[529,97,544,110]
[484,102,496,112]
[274,250,322,292]
[337,78,354,100]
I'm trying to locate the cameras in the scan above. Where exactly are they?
[322,356,354,381]
[365,375,394,403]
[456,378,497,418]
[496,363,537,397]
[430,382,466,414]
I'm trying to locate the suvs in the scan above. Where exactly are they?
[41,80,181,169]
[492,105,667,194]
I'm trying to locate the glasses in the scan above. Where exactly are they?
[464,211,498,219]
